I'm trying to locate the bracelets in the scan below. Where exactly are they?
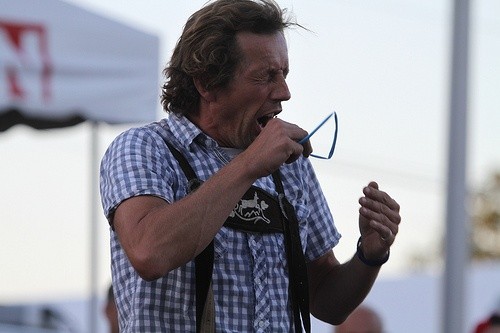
[356,236,390,267]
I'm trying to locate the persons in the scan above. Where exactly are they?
[97,0,403,333]
[103,282,500,333]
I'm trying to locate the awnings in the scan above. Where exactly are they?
[0,0,162,138]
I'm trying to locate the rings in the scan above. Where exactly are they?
[378,229,392,241]
